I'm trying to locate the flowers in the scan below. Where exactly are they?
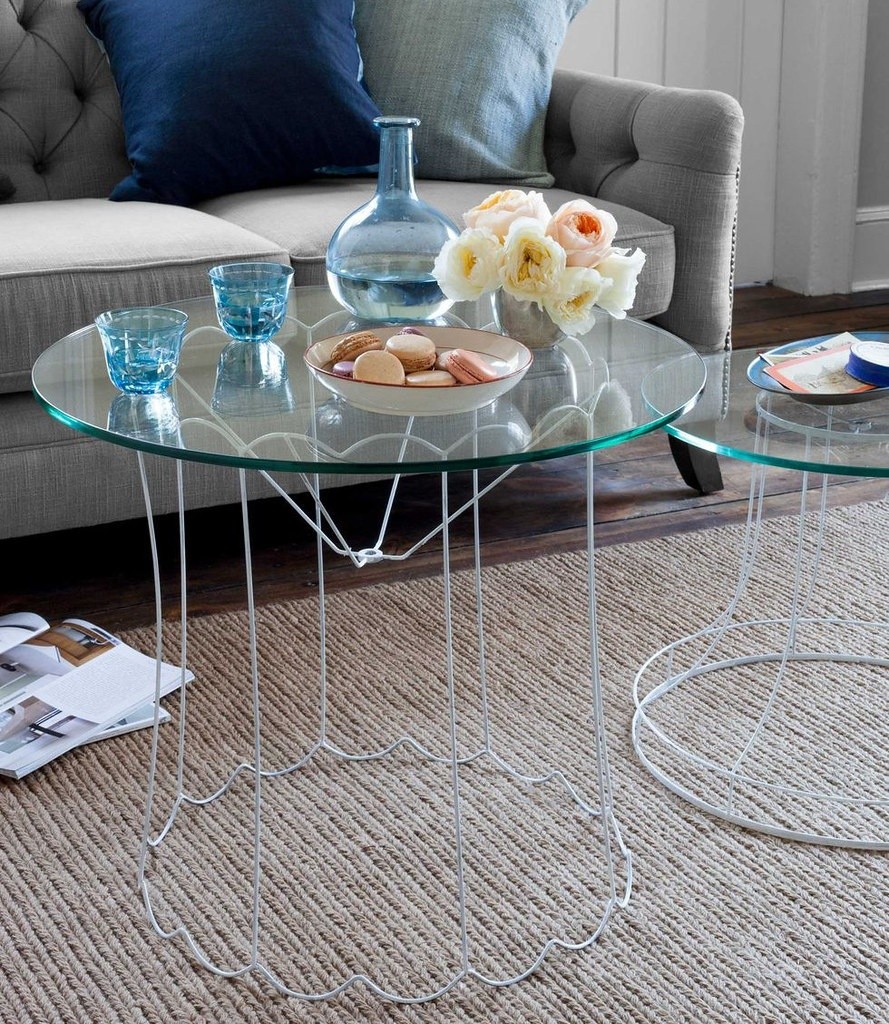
[429,189,647,337]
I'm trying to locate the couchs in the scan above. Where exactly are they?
[0,0,744,541]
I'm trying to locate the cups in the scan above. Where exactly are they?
[94,307,191,394]
[107,393,182,448]
[209,261,296,342]
[212,340,296,418]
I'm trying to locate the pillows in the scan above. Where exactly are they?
[76,0,419,203]
[352,0,591,188]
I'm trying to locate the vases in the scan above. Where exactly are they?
[491,287,568,349]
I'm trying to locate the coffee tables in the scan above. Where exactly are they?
[629,348,889,852]
[28,283,708,1004]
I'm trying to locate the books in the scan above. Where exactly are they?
[0,612,195,780]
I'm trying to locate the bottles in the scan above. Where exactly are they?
[325,115,464,323]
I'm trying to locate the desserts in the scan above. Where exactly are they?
[333,327,497,386]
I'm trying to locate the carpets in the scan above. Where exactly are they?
[0,498,889,1024]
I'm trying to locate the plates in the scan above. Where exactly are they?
[302,397,535,462]
[746,329,889,406]
[301,326,534,417]
